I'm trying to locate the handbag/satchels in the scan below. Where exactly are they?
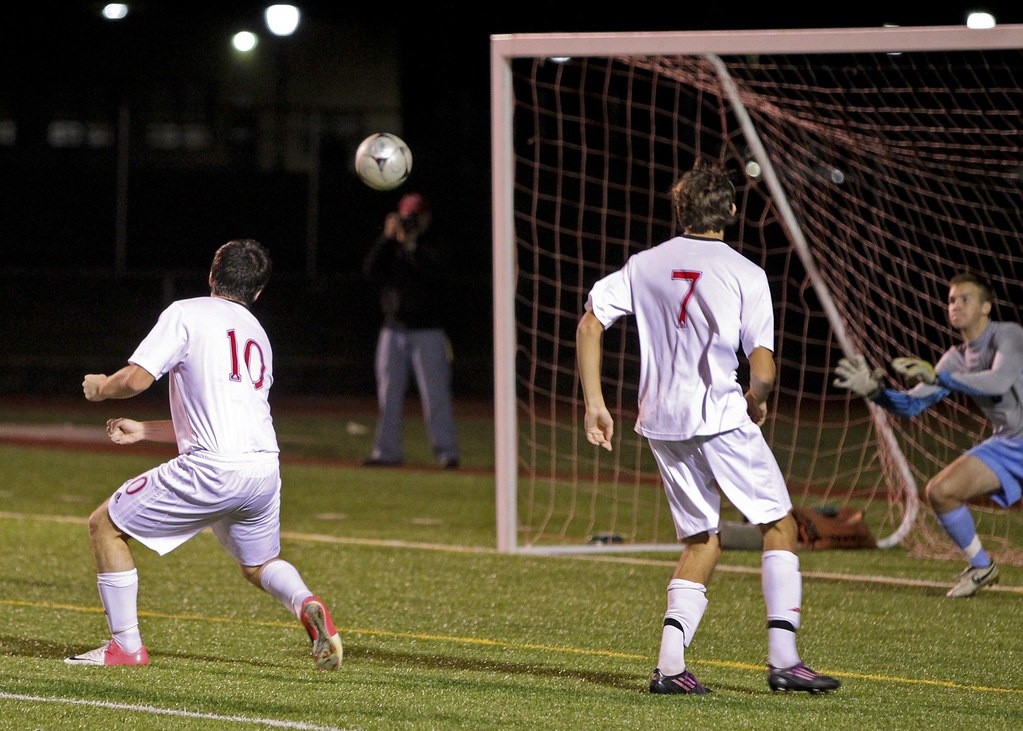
[791,505,876,551]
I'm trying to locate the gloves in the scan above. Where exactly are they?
[891,357,936,385]
[833,353,888,401]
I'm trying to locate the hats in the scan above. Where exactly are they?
[398,193,422,220]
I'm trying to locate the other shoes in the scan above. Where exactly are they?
[437,457,458,469]
[362,458,401,468]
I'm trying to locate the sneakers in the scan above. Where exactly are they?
[765,660,840,695]
[649,668,713,695]
[945,558,1000,598]
[63,639,149,666]
[294,591,343,671]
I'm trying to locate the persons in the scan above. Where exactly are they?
[832,273,1023,599]
[63,238,343,672]
[358,193,460,470]
[576,160,841,695]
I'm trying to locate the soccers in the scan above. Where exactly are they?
[353,131,413,192]
